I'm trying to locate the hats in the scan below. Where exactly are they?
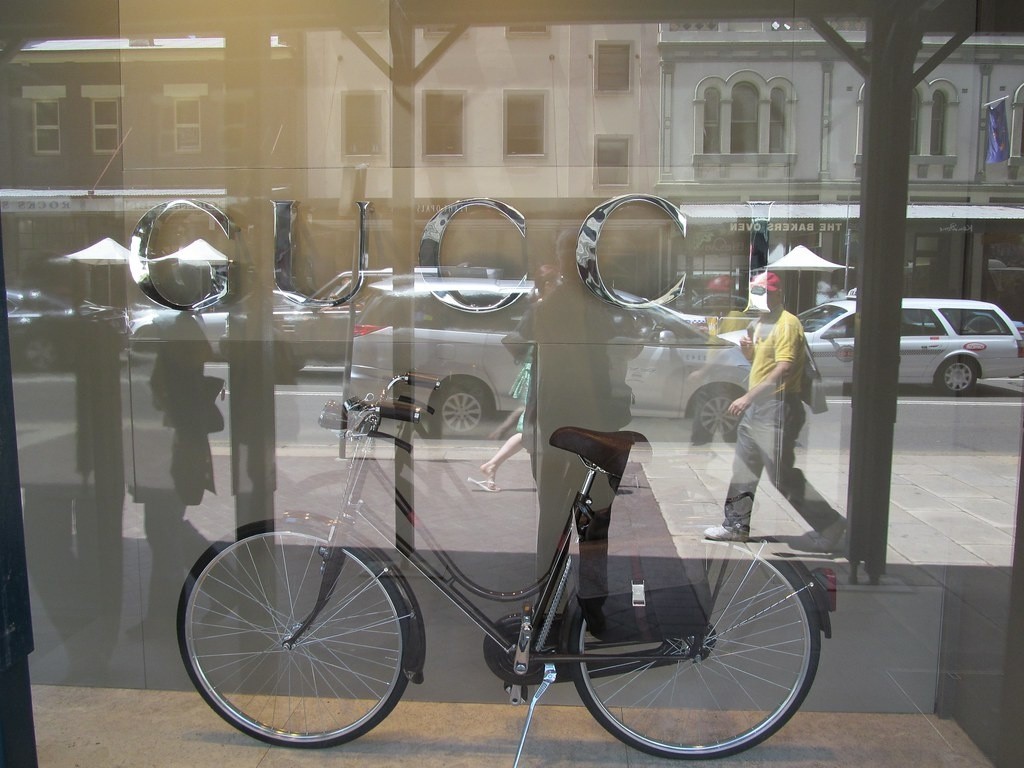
[708,276,733,291]
[747,272,781,293]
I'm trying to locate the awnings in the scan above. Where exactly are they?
[679,204,1022,219]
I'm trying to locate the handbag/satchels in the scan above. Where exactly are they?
[202,375,228,434]
[801,338,828,415]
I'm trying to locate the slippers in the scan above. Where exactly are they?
[467,476,500,492]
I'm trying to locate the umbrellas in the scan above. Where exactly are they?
[153,240,229,262]
[759,244,851,272]
[67,237,145,261]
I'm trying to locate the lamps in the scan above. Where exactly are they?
[769,18,809,31]
[665,21,719,32]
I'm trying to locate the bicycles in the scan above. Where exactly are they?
[175,368,842,768]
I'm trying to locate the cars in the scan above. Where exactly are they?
[344,274,758,444]
[130,288,303,354]
[2,286,130,374]
[712,295,1023,396]
[217,267,508,383]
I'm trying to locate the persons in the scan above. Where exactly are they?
[467,230,643,633]
[690,258,848,551]
[125,300,236,646]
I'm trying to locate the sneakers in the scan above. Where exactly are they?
[812,515,847,550]
[704,522,749,541]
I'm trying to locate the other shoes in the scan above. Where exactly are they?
[202,589,243,626]
[129,615,178,637]
